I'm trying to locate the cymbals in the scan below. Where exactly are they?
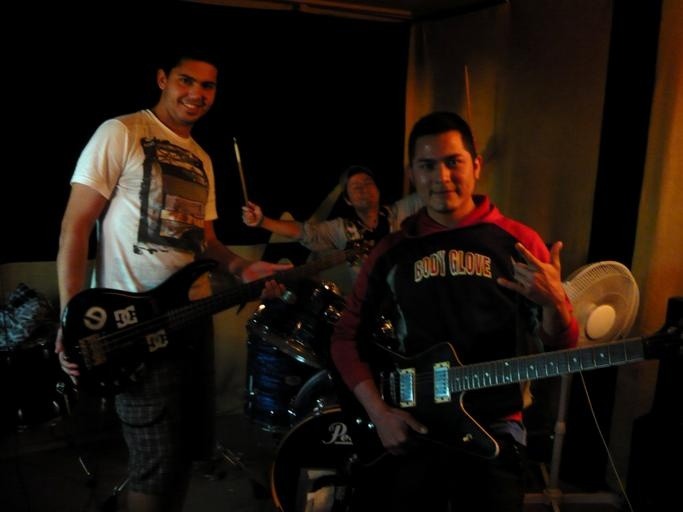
[301,265,351,313]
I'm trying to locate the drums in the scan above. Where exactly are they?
[271,362,381,510]
[295,279,346,325]
[246,330,336,433]
[245,292,331,369]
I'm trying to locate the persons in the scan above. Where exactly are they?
[241,125,480,277]
[327,110,580,512]
[57,48,295,512]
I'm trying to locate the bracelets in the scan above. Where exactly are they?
[257,216,265,228]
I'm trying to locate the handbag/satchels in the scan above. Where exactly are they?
[0,282,58,352]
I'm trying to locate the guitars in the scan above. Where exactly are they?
[331,296,682,483]
[61,238,377,396]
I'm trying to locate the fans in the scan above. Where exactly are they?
[549,261,640,491]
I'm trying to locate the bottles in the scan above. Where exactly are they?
[112,473,128,496]
[217,444,245,471]
